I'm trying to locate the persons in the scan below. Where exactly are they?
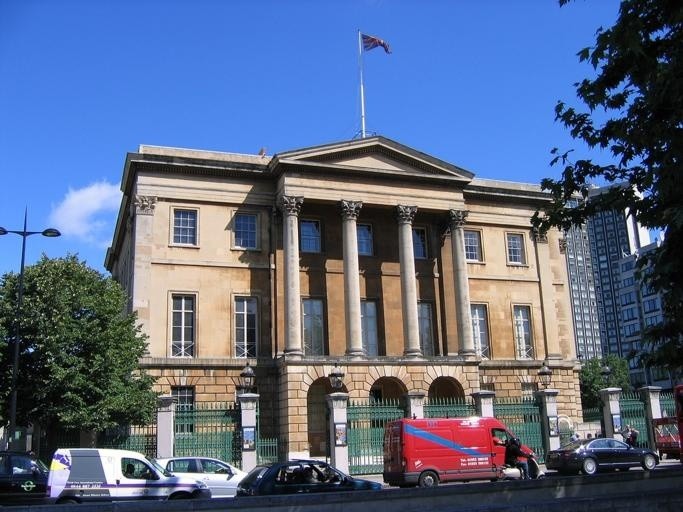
[125,464,136,478]
[305,467,335,483]
[492,432,505,445]
[621,424,639,447]
[504,439,532,480]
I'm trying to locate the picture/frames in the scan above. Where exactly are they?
[547,415,558,437]
[611,414,621,434]
[242,426,256,451]
[334,422,347,446]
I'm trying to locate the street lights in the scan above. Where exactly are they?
[0,205,61,451]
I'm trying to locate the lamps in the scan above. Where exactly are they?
[238,362,256,393]
[328,363,344,392]
[537,362,551,389]
[600,363,612,388]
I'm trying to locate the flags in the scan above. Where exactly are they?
[361,32,392,54]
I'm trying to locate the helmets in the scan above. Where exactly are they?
[510,438,521,447]
